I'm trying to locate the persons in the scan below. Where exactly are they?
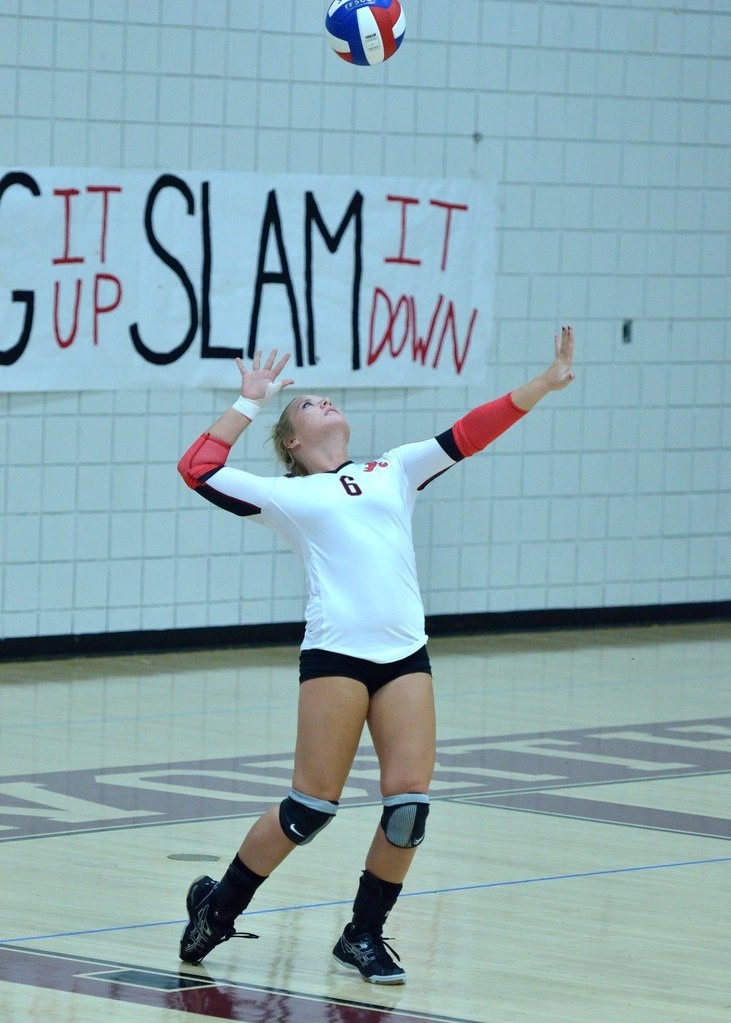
[175,326,576,984]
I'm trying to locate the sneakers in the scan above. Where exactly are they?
[177,874,260,966]
[331,922,406,985]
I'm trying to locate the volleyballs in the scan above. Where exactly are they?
[325,0,407,66]
